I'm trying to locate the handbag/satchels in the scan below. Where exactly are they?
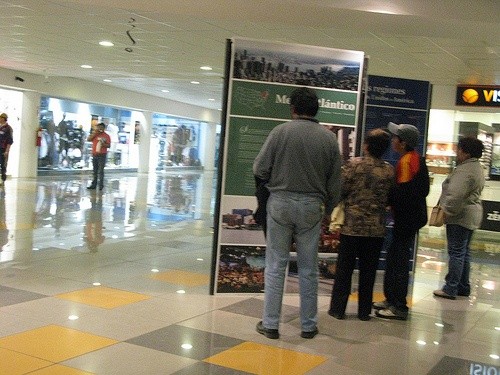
[428,206,444,227]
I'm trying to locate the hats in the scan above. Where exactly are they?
[388,121,420,144]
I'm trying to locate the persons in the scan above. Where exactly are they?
[86,123,110,191]
[433,137,484,300]
[253,87,339,339]
[58,112,69,153]
[0,113,13,186]
[173,125,189,164]
[39,120,85,169]
[371,121,430,321]
[328,128,396,320]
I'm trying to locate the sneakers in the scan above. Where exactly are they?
[255,322,280,339]
[301,329,318,339]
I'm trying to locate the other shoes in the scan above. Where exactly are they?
[328,309,346,319]
[376,306,408,320]
[372,298,390,308]
[433,288,457,300]
[358,313,370,321]
